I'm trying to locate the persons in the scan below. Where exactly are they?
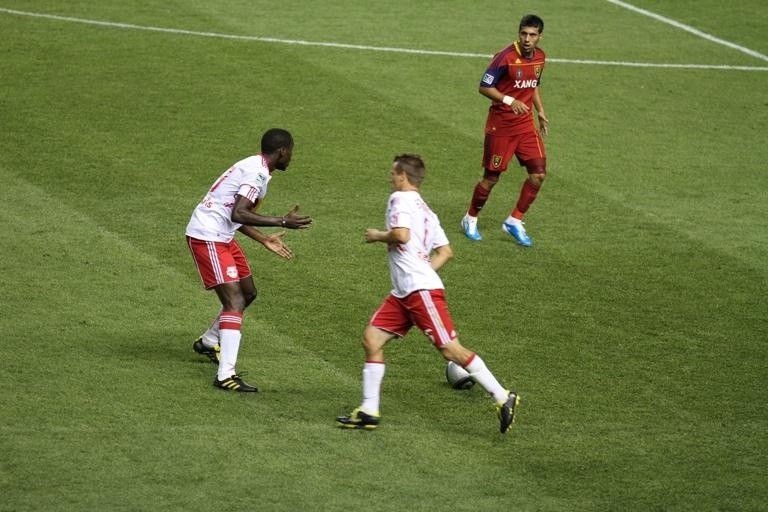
[460,15,549,247]
[335,154,520,434]
[184,129,313,393]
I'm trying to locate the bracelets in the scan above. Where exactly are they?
[502,95,515,106]
[282,216,286,227]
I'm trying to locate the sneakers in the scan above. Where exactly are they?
[502,221,532,246]
[335,409,378,429]
[461,214,480,240]
[193,336,221,365]
[213,374,258,393]
[496,393,519,434]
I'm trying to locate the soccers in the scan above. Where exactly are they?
[446,360,476,389]
